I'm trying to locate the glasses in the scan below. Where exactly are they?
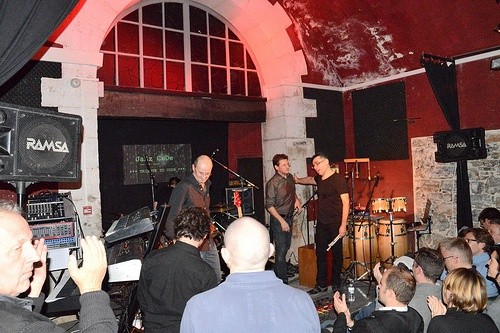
[311,159,327,169]
[480,221,485,225]
[443,255,459,260]
[465,237,477,243]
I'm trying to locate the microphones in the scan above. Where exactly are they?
[211,149,218,157]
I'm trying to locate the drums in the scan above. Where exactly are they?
[341,219,354,278]
[391,197,407,213]
[370,197,390,213]
[348,219,378,280]
[378,218,409,269]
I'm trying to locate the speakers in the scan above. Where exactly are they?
[0,102,82,184]
[432,126,488,162]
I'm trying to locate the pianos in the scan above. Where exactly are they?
[40,206,154,333]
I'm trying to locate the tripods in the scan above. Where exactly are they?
[339,168,375,294]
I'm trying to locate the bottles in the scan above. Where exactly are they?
[348,284,355,301]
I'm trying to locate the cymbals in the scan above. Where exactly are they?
[212,204,227,207]
[210,206,236,213]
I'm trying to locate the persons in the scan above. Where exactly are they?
[163,178,182,208]
[178,216,322,333]
[0,195,121,333]
[265,152,302,286]
[164,153,222,286]
[292,154,350,294]
[321,206,500,333]
[136,208,216,333]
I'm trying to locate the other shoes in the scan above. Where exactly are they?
[307,286,328,294]
[286,272,295,277]
[333,288,342,295]
